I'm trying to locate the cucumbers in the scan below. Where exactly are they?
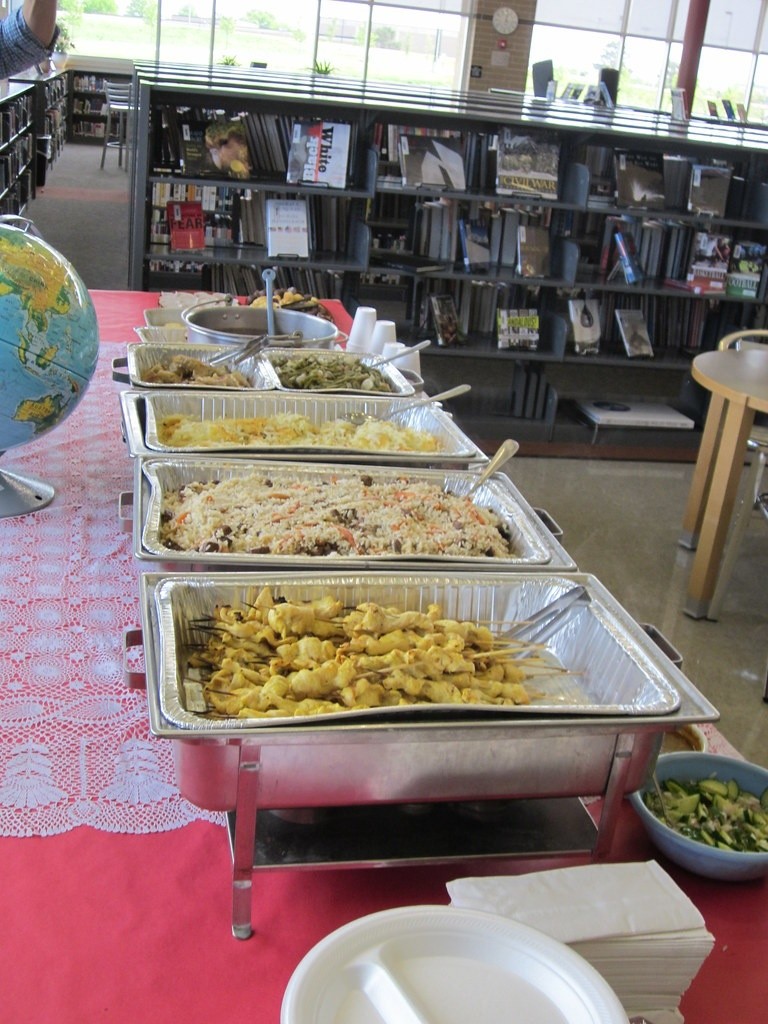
[644,778,768,856]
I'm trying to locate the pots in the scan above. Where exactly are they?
[181,296,348,347]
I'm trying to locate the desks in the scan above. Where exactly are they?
[679,349,768,624]
[0,289,768,1024]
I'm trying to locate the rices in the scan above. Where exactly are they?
[158,474,510,562]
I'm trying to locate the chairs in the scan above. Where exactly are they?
[706,329,768,629]
[100,79,135,172]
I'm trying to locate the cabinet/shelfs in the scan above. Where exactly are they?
[67,54,135,146]
[9,66,68,186]
[0,81,37,225]
[129,80,768,468]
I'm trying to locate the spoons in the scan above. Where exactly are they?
[341,384,471,424]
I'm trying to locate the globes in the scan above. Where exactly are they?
[0,214,101,518]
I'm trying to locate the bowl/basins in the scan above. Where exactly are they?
[629,750,768,879]
[281,905,630,1024]
[656,723,710,754]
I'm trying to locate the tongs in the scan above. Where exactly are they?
[209,336,270,367]
[500,587,591,658]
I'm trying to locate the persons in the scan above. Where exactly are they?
[0,0,62,80]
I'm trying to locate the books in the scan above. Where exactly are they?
[152,102,768,361]
[0,73,126,219]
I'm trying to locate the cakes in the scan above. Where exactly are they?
[243,287,328,322]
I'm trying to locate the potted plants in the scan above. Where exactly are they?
[52,23,75,68]
